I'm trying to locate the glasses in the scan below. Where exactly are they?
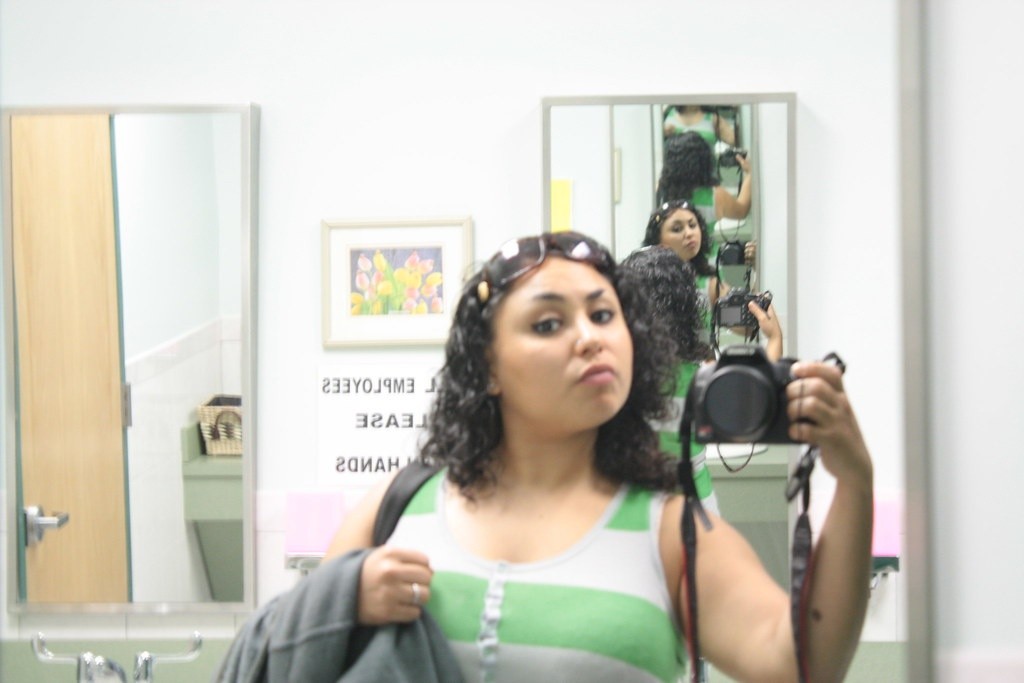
[476,232,615,313]
[651,200,690,228]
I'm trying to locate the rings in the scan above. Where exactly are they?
[412,583,420,604]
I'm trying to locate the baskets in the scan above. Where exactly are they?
[197,395,242,456]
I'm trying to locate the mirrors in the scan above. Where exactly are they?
[608,103,761,294]
[536,93,797,409]
[0,0,939,683]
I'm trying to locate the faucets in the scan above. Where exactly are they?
[82,653,123,679]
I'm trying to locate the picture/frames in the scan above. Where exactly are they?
[317,219,477,353]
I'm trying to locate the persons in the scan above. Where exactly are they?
[616,246,783,517]
[643,105,757,360]
[320,231,875,683]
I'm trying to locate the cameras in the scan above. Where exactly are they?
[718,147,744,168]
[715,239,746,266]
[710,285,759,327]
[691,345,815,445]
